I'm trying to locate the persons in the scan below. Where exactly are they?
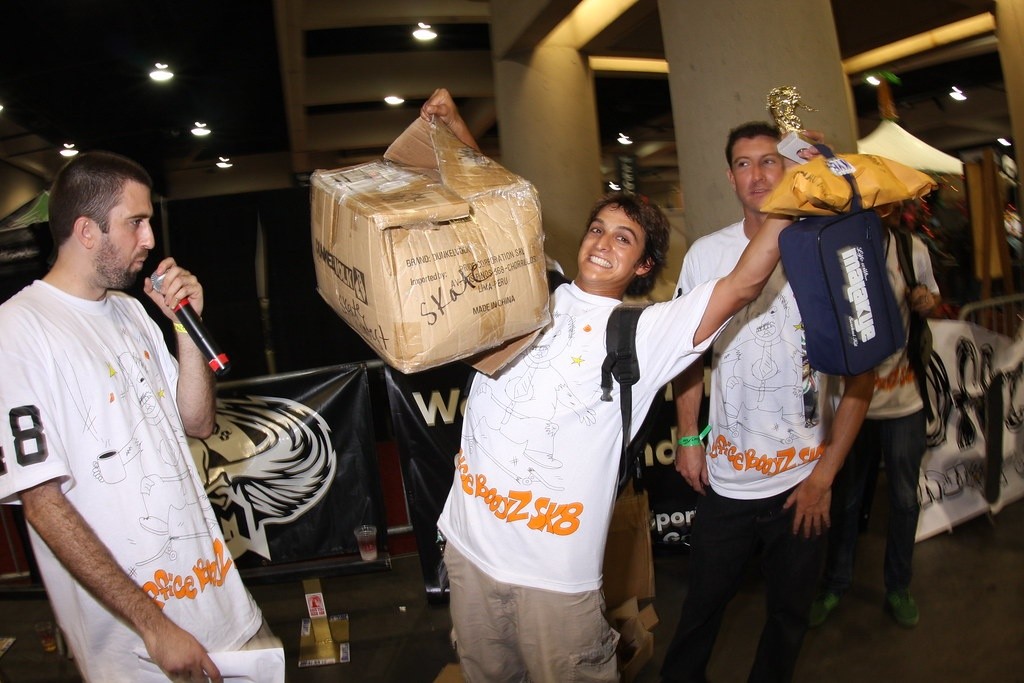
[826,208,947,626]
[0,152,288,683]
[422,88,832,683]
[652,121,874,683]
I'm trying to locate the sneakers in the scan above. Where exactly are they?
[808,593,838,627]
[885,585,920,625]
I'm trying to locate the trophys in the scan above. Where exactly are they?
[765,84,821,167]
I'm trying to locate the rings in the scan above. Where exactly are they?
[925,296,928,302]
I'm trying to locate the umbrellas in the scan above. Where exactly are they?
[860,112,963,177]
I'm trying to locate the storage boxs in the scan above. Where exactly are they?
[603,479,656,607]
[606,595,660,683]
[311,114,551,376]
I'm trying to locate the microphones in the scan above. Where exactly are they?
[150,270,232,377]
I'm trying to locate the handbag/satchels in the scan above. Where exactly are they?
[778,142,906,375]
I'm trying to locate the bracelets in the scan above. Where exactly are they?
[930,292,941,316]
[173,318,203,333]
[677,426,713,447]
